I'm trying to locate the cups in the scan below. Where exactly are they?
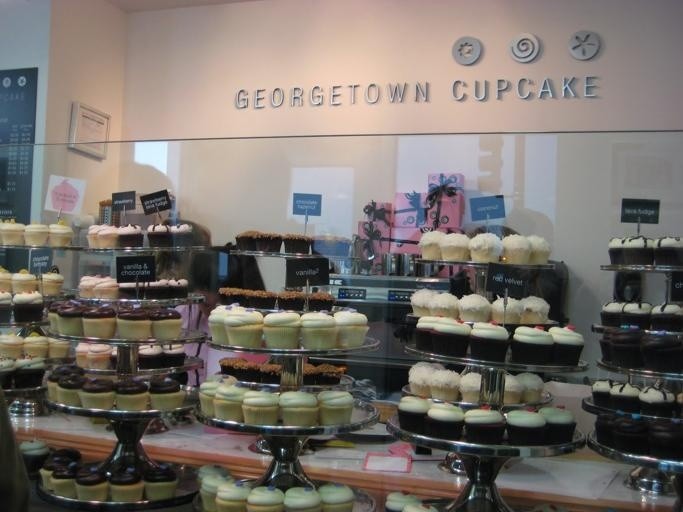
[381,252,401,276]
[399,253,421,277]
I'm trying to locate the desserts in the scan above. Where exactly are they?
[0,220,683,511]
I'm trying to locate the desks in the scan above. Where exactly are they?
[8,407,682,511]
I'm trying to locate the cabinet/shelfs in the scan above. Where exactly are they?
[309,262,450,392]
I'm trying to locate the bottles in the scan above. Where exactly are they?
[347,234,360,275]
[22,398,40,419]
[6,398,24,418]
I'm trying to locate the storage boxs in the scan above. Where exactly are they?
[358,173,466,278]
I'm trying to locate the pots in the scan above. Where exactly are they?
[332,256,361,275]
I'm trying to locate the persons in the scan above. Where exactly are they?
[0,386,32,512]
[153,219,219,388]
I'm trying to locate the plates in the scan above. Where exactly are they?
[333,419,399,443]
[386,441,449,461]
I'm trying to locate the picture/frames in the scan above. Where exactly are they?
[67,101,112,159]
